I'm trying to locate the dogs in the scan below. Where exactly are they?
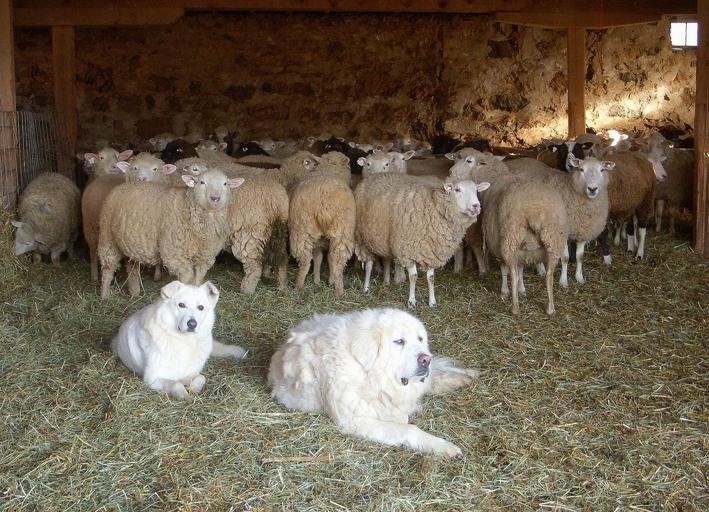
[263,306,479,460]
[109,278,250,401]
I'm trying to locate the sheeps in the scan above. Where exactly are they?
[7,170,83,268]
[73,126,698,317]
